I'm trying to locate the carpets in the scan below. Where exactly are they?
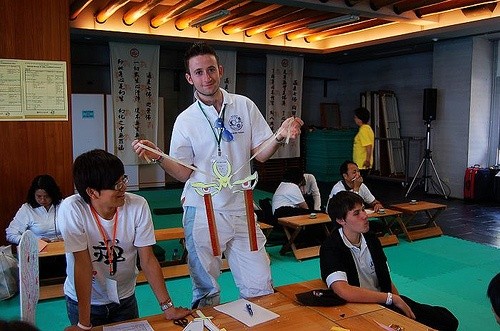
[0,189,500,331]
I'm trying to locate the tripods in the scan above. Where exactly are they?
[404,123,449,200]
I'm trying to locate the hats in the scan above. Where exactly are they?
[295,289,346,306]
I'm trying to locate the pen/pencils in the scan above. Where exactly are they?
[246,304,254,316]
[350,178,356,182]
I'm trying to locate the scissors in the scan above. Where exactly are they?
[173,318,188,328]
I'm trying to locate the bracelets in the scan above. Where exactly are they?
[78,322,92,331]
[161,302,173,310]
[155,156,162,161]
[274,133,285,144]
[386,293,392,304]
[160,298,171,305]
[162,154,167,160]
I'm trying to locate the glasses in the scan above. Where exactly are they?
[214,118,234,142]
[104,174,129,190]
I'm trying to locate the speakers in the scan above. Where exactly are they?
[422,88,437,121]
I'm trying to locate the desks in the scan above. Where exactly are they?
[366,208,402,246]
[278,213,331,260]
[38,242,67,299]
[92,280,439,331]
[391,202,447,240]
[136,227,190,284]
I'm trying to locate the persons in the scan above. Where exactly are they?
[132,44,304,308]
[6,175,66,278]
[487,272,500,325]
[57,150,197,331]
[353,108,374,181]
[321,191,459,331]
[326,161,383,212]
[271,169,321,255]
